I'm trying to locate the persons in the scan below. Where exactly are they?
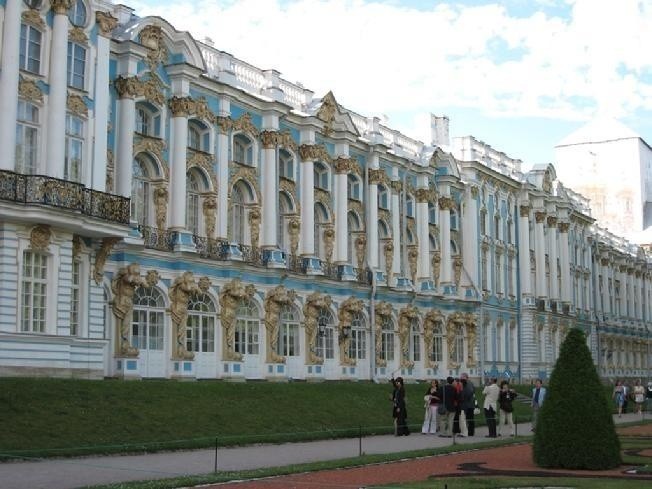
[390,373,410,436]
[496,380,520,438]
[481,377,500,439]
[528,379,547,432]
[420,371,479,438]
[612,379,652,418]
